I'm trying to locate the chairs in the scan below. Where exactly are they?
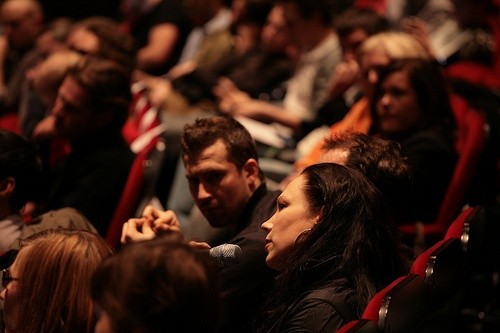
[0,17,500,333]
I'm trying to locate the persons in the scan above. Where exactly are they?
[0,0,500,333]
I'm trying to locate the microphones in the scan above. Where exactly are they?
[194,242,243,268]
